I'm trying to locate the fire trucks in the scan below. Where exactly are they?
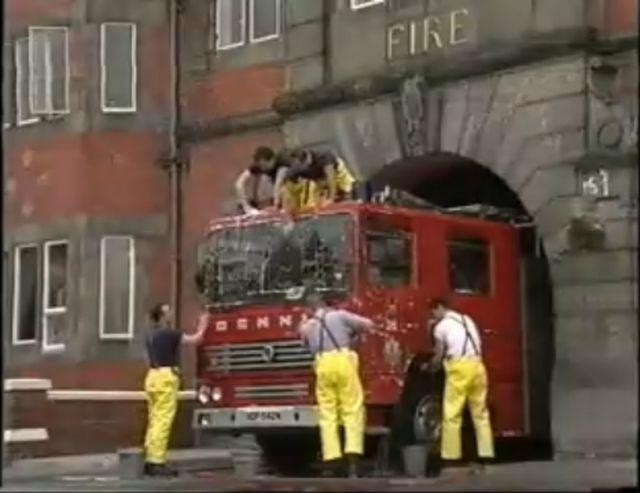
[189,185,550,463]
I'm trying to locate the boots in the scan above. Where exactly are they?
[320,452,364,479]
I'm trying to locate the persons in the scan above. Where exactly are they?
[234,145,355,214]
[143,302,210,477]
[300,293,377,479]
[425,296,496,468]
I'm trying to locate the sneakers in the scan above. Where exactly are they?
[143,460,179,478]
